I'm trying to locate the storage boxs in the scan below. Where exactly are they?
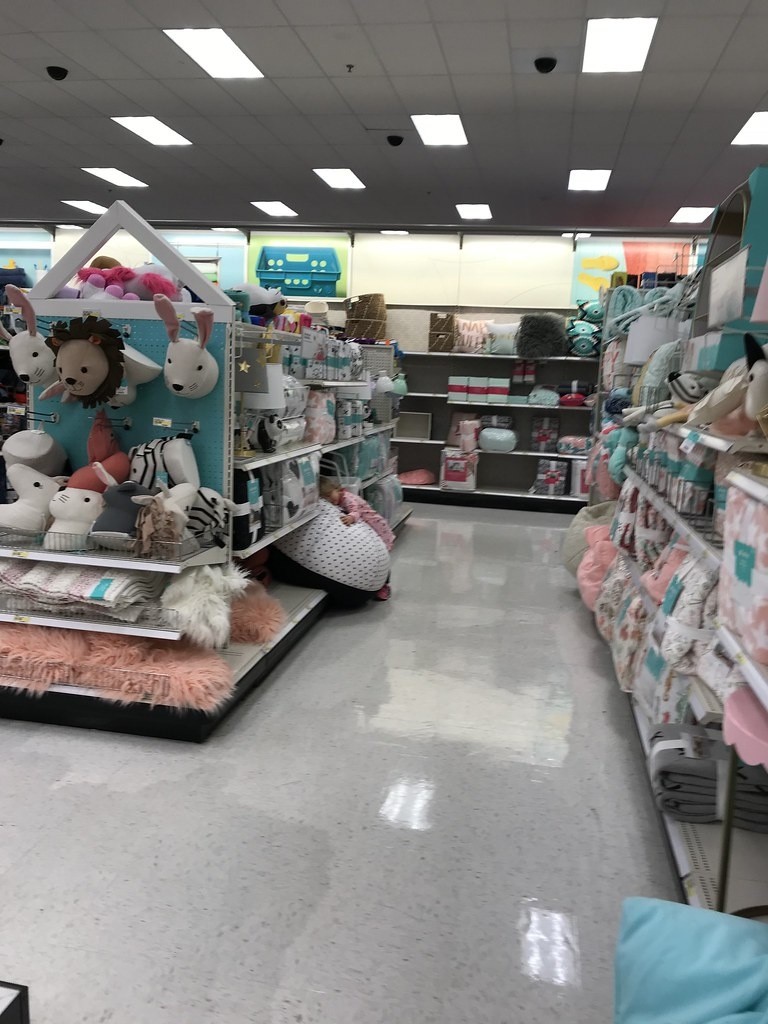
[393,312,590,498]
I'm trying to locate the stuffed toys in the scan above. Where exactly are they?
[0,255,290,559]
[620,333,768,435]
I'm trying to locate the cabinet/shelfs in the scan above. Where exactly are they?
[0,240,415,743]
[593,390,768,914]
[392,351,598,501]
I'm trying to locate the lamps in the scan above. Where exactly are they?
[231,346,269,456]
[369,368,395,394]
[389,372,409,395]
[245,362,287,410]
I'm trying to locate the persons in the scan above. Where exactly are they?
[320,476,398,600]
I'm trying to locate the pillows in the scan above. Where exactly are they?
[485,322,521,355]
[450,318,494,354]
